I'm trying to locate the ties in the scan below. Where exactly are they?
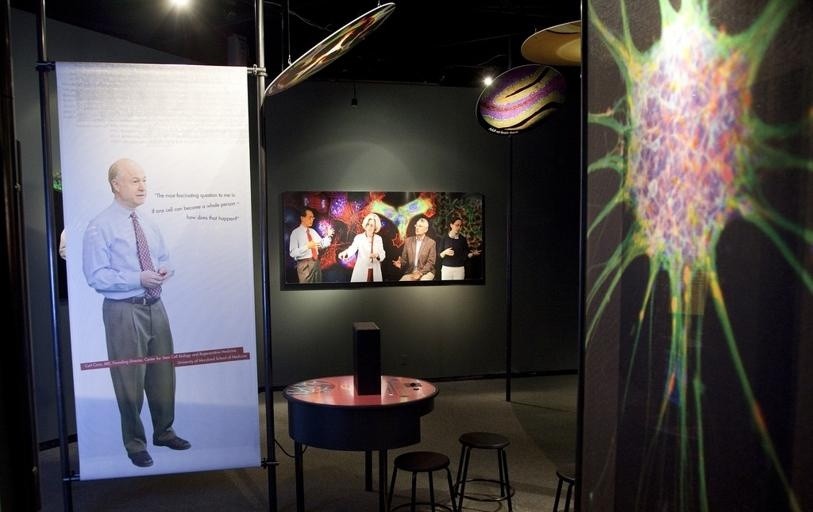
[130,212,163,299]
[306,229,317,260]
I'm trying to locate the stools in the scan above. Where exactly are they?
[552,468,576,512]
[453,432,515,512]
[387,451,457,512]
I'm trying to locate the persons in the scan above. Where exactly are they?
[338,213,385,282]
[59,229,67,260]
[438,217,482,280]
[392,218,436,281]
[83,159,191,466]
[289,210,335,283]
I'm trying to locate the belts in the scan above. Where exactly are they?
[129,297,159,304]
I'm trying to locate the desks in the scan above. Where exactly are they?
[282,373,440,512]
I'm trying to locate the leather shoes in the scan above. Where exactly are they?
[127,451,153,467]
[153,436,191,450]
